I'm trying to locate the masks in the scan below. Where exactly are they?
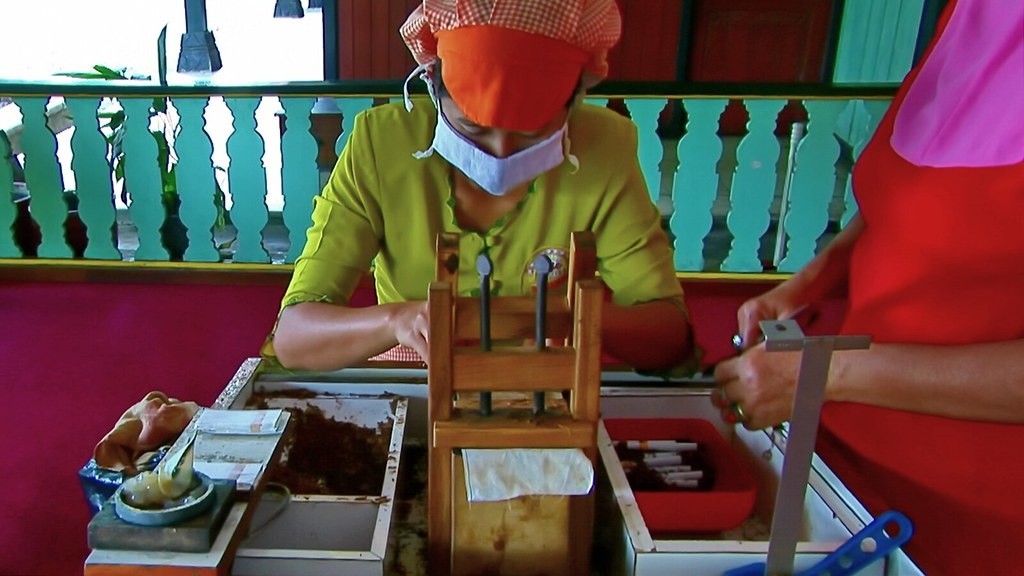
[411,97,580,194]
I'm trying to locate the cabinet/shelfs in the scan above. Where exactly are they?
[83,357,927,576]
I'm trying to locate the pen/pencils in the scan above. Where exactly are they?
[612,436,704,491]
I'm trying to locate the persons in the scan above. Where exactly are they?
[711,0,1024,576]
[274,1,697,370]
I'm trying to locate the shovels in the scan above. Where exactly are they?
[719,511,915,576]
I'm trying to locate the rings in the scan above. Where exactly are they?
[735,402,747,422]
[731,336,742,346]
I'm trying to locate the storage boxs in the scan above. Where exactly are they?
[605,418,755,532]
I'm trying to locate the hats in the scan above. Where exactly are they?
[399,0,621,132]
